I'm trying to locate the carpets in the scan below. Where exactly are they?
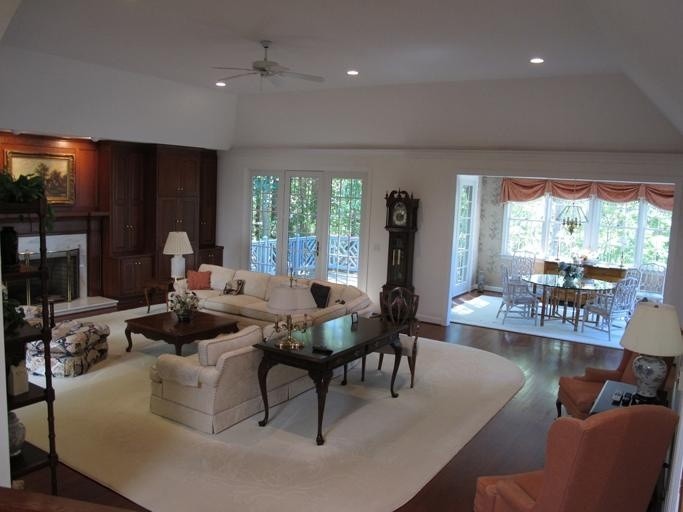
[9,302,527,512]
[448,293,632,350]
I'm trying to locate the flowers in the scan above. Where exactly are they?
[169,288,200,312]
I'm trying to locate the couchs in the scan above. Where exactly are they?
[148,263,375,435]
[14,304,111,379]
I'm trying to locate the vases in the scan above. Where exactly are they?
[174,311,194,324]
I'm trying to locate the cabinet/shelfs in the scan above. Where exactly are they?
[0,195,58,496]
[97,140,225,311]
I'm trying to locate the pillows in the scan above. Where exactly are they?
[264,315,314,341]
[198,324,263,366]
[186,263,370,323]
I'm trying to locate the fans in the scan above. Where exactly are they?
[209,40,325,94]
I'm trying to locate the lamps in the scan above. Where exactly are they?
[162,231,195,279]
[555,181,589,236]
[263,267,318,350]
[619,301,683,404]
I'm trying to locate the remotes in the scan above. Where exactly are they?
[611,390,623,402]
[621,392,632,404]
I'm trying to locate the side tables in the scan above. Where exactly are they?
[588,379,668,417]
[135,279,176,315]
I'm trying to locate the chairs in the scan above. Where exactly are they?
[495,251,667,342]
[555,348,675,421]
[473,404,680,512]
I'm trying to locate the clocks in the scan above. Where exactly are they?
[380,187,421,325]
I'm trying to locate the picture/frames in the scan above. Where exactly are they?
[3,147,76,208]
